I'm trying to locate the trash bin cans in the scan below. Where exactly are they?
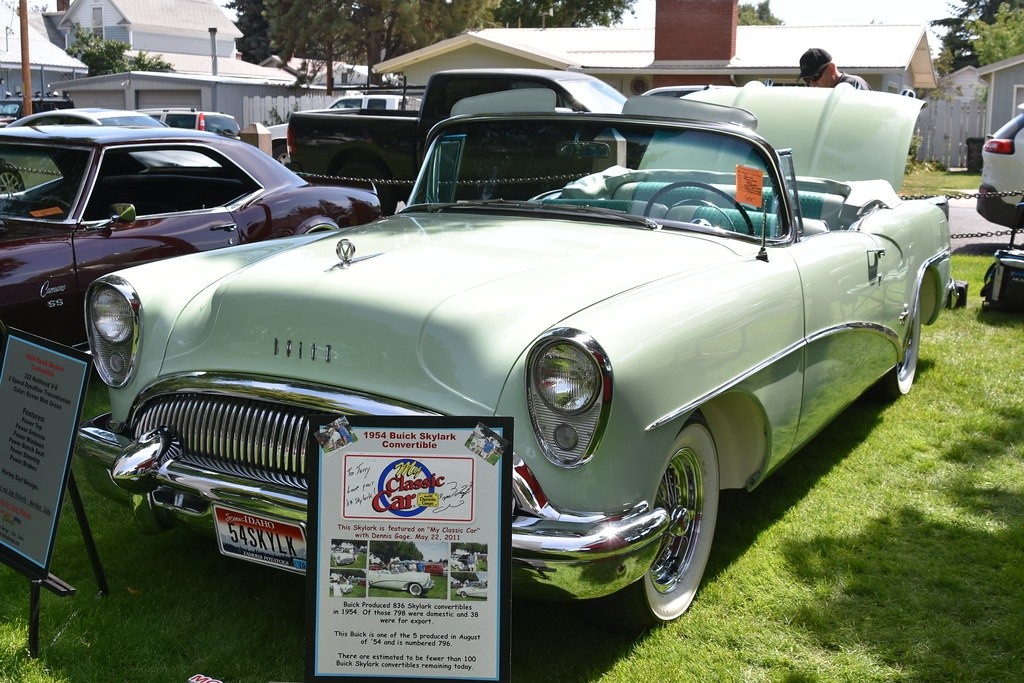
[966,137,985,173]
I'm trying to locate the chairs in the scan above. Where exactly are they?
[533,199,670,219]
[664,205,830,237]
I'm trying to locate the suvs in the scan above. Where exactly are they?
[265,95,422,171]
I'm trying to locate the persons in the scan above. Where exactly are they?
[795,48,874,91]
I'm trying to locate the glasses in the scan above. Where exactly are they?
[803,68,828,84]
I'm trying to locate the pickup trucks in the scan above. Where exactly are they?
[285,66,631,216]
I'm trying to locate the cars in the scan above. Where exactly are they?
[71,81,952,631]
[975,101,1024,230]
[0,97,381,374]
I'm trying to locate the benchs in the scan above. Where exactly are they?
[100,174,245,207]
[610,180,845,220]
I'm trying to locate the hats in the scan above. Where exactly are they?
[795,48,833,83]
